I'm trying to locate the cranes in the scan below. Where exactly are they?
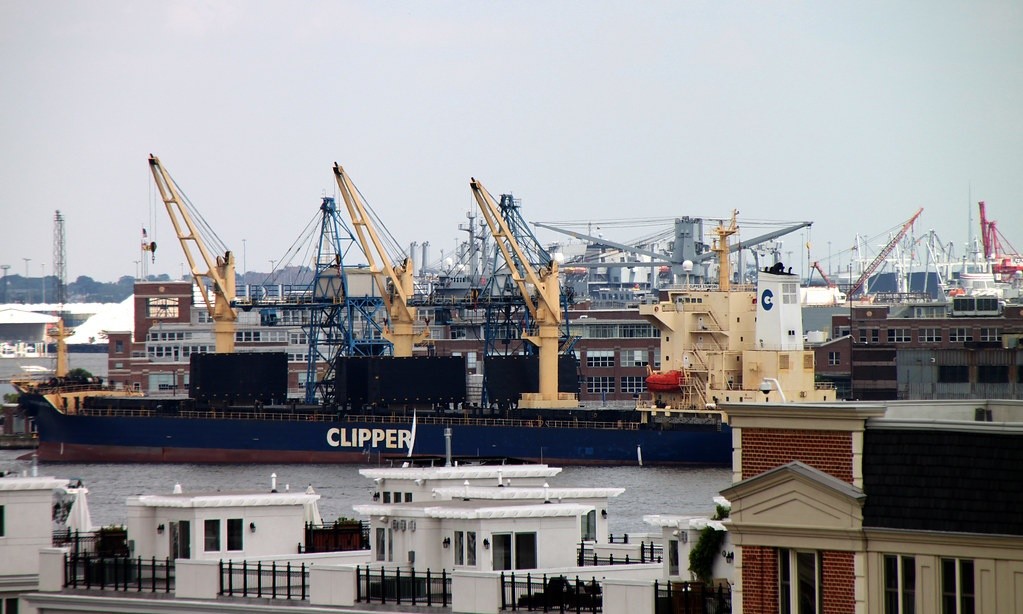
[141,152,1022,413]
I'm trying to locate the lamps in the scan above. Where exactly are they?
[443,537,451,548]
[726,551,734,564]
[601,510,607,519]
[483,539,490,549]
[250,522,256,533]
[759,376,787,404]
[373,492,380,501]
[156,524,165,534]
